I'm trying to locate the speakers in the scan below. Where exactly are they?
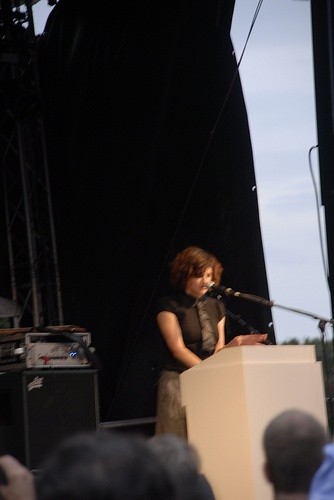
[11,370,101,471]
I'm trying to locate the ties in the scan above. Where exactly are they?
[196,302,216,351]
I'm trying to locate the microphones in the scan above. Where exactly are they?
[206,281,241,296]
